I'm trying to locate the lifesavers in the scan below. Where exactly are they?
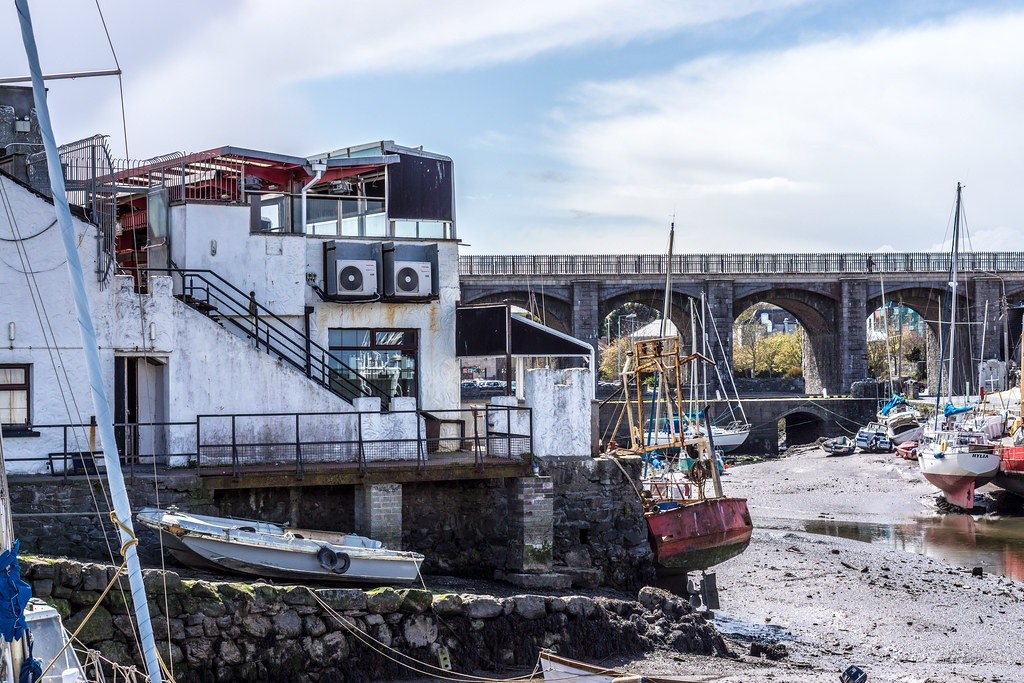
[317,548,351,574]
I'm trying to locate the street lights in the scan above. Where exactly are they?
[618,313,636,381]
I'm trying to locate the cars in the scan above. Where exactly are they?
[598,380,621,386]
[461,381,507,398]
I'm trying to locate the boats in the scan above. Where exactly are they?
[822,436,919,459]
[916,182,1024,511]
[876,271,923,446]
[599,214,753,587]
[136,505,425,585]
